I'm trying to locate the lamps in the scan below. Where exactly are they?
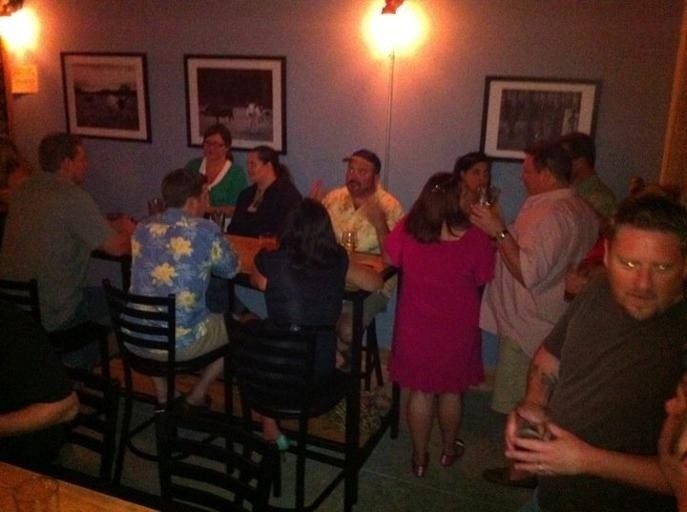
[361,0,429,194]
[2,5,43,83]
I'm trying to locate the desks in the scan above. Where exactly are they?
[89,233,401,506]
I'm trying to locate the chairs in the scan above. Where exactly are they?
[58,364,122,496]
[100,277,242,499]
[220,310,357,512]
[149,401,282,512]
[0,277,111,378]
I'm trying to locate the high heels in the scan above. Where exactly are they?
[439,441,463,468]
[413,453,430,477]
[267,434,290,460]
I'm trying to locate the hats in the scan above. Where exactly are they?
[342,148,381,167]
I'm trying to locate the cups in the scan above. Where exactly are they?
[148,198,162,217]
[212,211,226,229]
[341,231,357,252]
[514,403,553,466]
[479,185,501,208]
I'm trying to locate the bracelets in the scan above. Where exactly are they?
[492,229,510,243]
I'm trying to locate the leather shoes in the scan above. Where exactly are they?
[484,465,539,490]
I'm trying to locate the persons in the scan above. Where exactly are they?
[468,139,605,489]
[121,168,243,417]
[1,303,82,471]
[309,149,407,372]
[382,172,496,478]
[226,145,304,333]
[564,128,618,221]
[229,198,350,463]
[504,193,687,512]
[421,151,502,247]
[1,133,31,204]
[0,131,139,366]
[658,374,687,512]
[185,123,248,234]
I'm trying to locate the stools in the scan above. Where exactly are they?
[359,318,385,393]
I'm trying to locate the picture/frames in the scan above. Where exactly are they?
[181,51,289,158]
[57,50,155,146]
[478,73,603,166]
